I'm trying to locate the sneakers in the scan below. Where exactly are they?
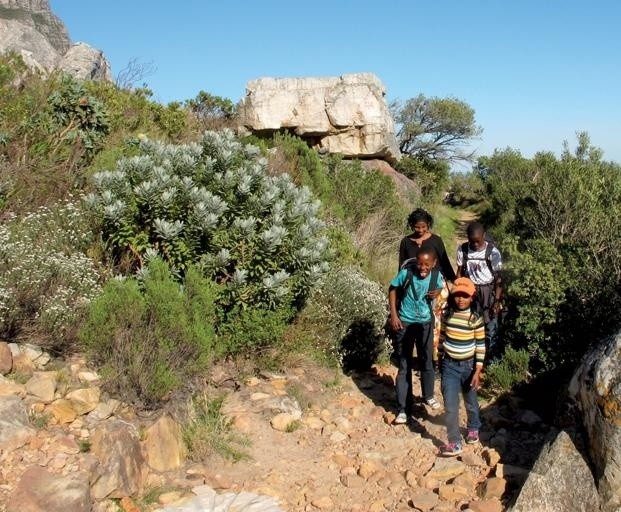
[442,441,462,456]
[395,412,409,424]
[466,430,480,444]
[427,398,441,410]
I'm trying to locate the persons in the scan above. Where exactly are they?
[437,276,488,459]
[388,247,442,424]
[398,208,455,375]
[457,221,507,357]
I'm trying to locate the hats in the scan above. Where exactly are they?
[451,277,476,296]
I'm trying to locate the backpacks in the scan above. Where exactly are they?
[400,257,440,302]
